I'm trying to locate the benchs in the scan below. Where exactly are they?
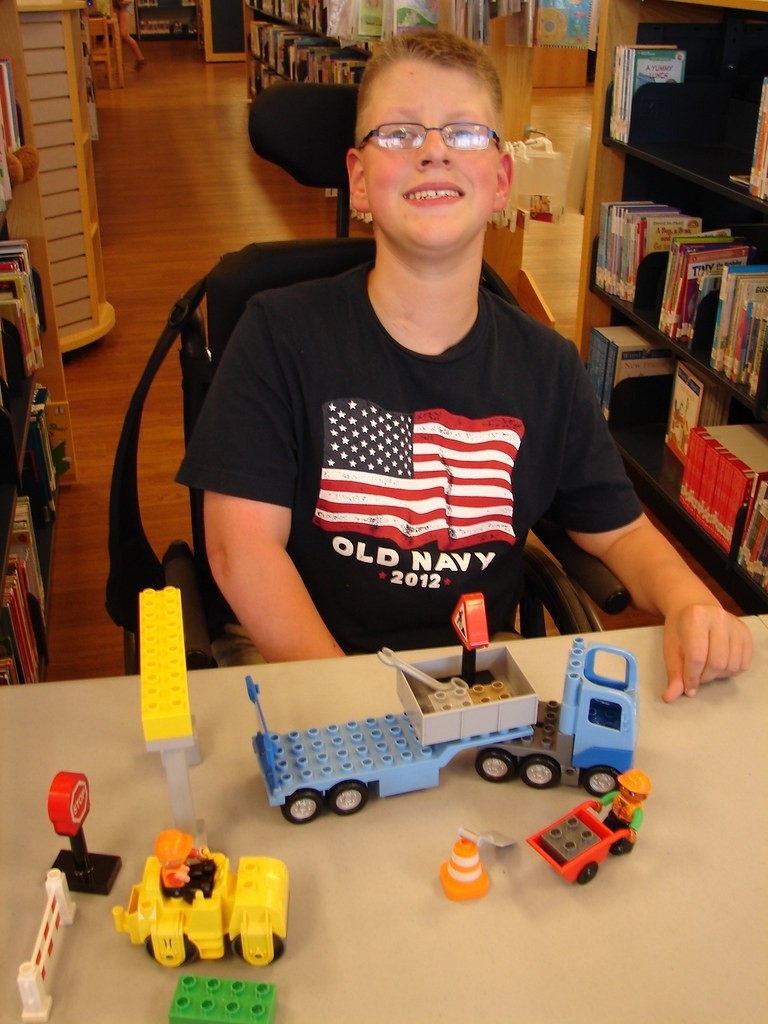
[87,14,124,88]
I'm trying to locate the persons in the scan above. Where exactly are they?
[595,770,650,854]
[154,829,215,898]
[111,0,146,72]
[173,28,752,701]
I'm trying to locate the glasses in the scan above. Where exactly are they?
[359,121,501,151]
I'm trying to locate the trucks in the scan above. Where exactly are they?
[242,633,640,827]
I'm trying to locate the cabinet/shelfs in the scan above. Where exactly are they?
[243,0,768,628]
[0,1,78,684]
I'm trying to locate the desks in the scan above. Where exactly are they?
[0,612,768,1024]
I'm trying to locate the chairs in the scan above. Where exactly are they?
[87,17,114,90]
[103,80,629,675]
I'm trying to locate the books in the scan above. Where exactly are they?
[590,44,768,590]
[138,0,196,34]
[0,58,78,685]
[81,19,99,139]
[245,0,438,98]
